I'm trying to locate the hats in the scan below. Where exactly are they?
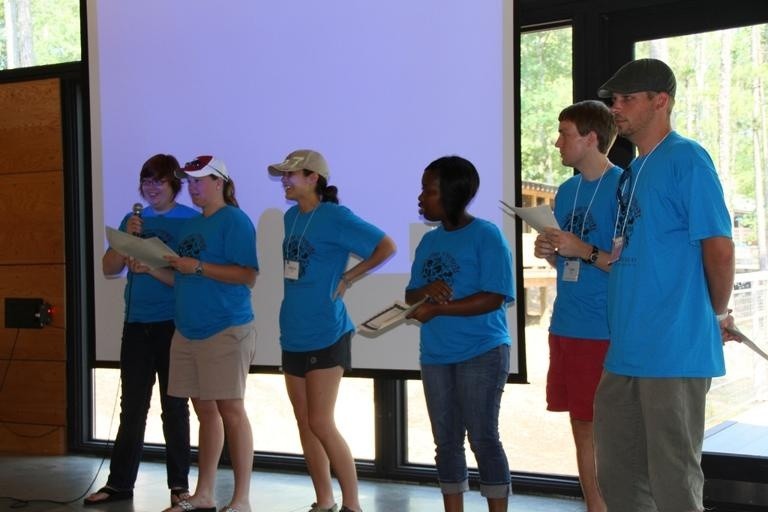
[596,58,677,99]
[267,149,329,182]
[173,155,229,186]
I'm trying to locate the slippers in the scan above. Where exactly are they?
[161,498,251,512]
[83,487,133,506]
[170,488,190,508]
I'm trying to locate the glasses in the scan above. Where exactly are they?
[185,159,228,180]
[139,178,174,187]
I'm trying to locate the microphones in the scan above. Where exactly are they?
[133,203,143,236]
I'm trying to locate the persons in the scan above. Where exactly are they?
[403,154,516,512]
[82,152,203,506]
[267,147,397,512]
[593,58,742,512]
[533,99,626,512]
[120,156,267,512]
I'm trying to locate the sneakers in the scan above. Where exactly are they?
[307,502,365,512]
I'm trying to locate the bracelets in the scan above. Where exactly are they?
[195,260,204,276]
[713,308,734,324]
[340,270,357,289]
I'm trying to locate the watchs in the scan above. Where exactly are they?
[582,248,600,264]
